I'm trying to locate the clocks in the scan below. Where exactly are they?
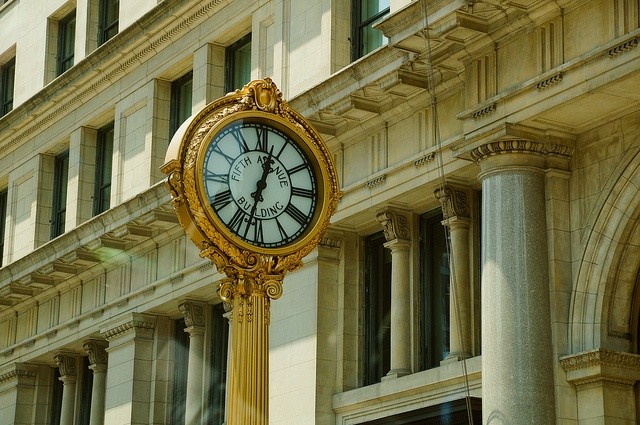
[158,76,344,302]
